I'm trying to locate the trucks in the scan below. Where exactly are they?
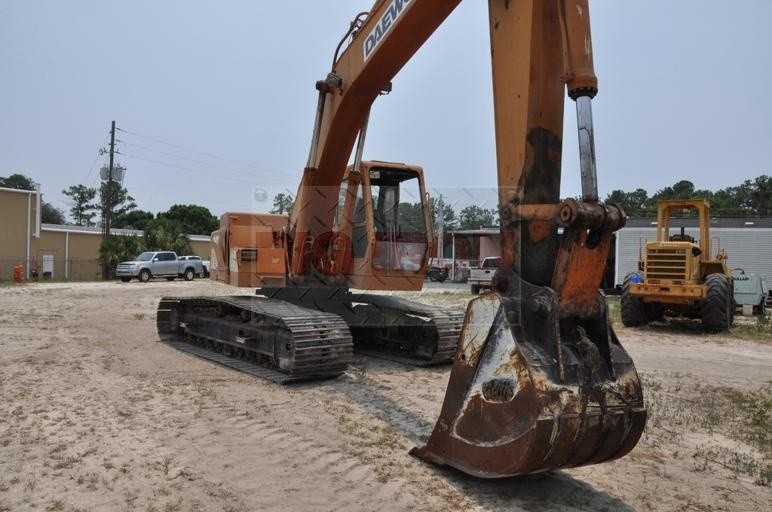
[468,256,502,294]
[156,0,648,480]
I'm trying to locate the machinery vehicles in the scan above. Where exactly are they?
[620,198,734,332]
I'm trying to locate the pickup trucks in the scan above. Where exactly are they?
[115,250,210,282]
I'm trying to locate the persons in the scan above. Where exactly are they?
[359,196,388,254]
